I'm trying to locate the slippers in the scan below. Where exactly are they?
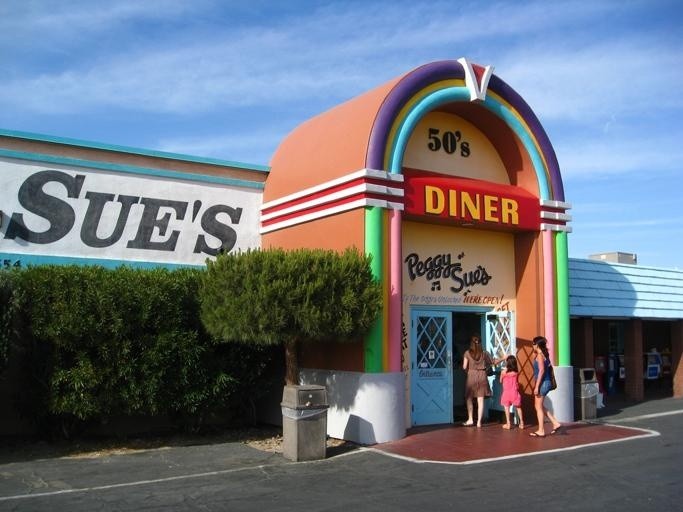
[528,432,546,438]
[550,426,561,436]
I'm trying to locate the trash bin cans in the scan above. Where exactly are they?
[280,384,329,462]
[573,367,599,421]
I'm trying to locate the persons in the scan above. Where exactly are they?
[528,335,562,437]
[498,355,524,431]
[458,334,493,427]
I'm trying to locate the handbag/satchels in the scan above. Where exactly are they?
[486,362,493,377]
[548,364,557,390]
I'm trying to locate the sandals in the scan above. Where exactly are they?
[518,423,525,429]
[462,420,473,426]
[477,423,482,428]
[502,424,511,430]
